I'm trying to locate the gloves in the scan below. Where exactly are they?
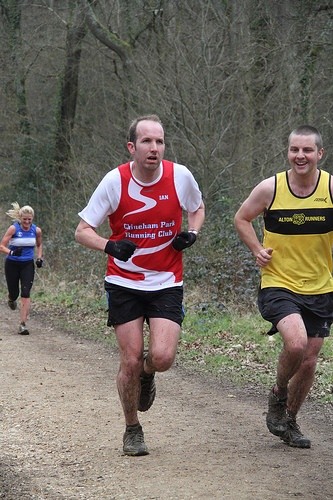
[104,239,139,262]
[172,231,197,252]
[9,248,22,257]
[35,258,43,268]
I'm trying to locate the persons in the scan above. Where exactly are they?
[234,125,333,447]
[0,202,43,335]
[75,115,205,456]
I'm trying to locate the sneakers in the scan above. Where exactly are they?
[279,414,311,449]
[17,321,30,335]
[122,424,149,457]
[8,300,18,310]
[138,350,157,412]
[266,386,288,436]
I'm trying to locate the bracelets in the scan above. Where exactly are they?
[188,231,197,236]
